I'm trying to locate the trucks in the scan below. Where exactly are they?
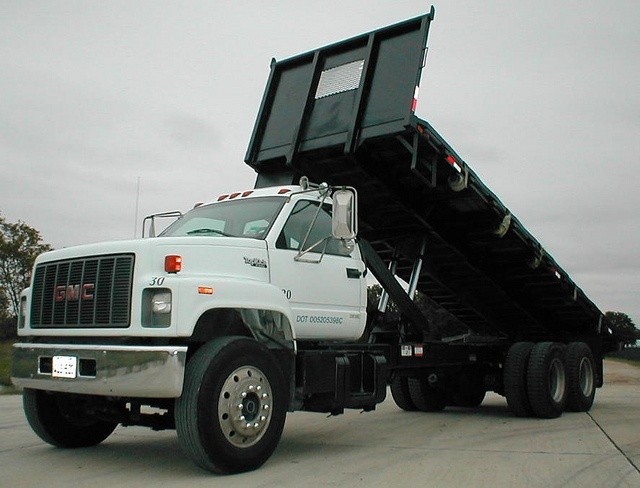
[10,6,622,475]
